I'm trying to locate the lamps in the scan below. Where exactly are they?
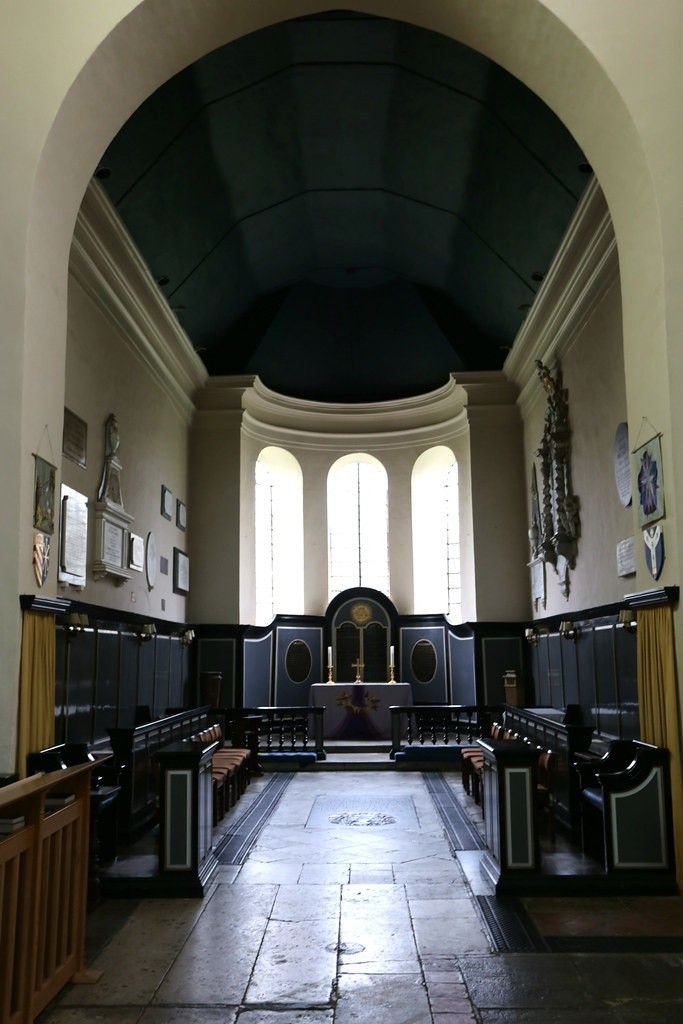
[65,611,90,636]
[182,629,194,648]
[138,624,157,642]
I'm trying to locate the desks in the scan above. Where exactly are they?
[308,681,418,739]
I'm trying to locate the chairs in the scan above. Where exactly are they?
[182,723,252,826]
[573,738,672,872]
[461,721,554,826]
[33,741,124,872]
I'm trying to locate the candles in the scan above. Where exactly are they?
[390,646,395,666]
[327,646,332,666]
